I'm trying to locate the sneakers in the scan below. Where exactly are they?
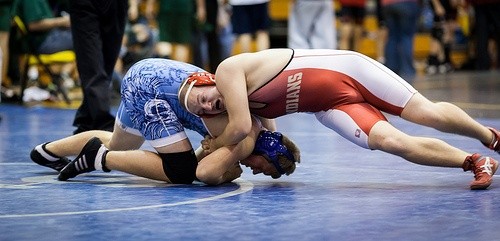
[481,126,500,152]
[462,152,499,191]
[57,136,109,181]
[30,140,72,173]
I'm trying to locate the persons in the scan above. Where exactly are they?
[288,0,337,49]
[69,0,131,135]
[216,0,275,54]
[425,0,454,75]
[0,0,24,105]
[30,58,301,186]
[145,0,206,64]
[380,0,420,85]
[177,48,500,190]
[21,0,123,99]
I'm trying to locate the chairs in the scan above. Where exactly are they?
[13,15,76,105]
